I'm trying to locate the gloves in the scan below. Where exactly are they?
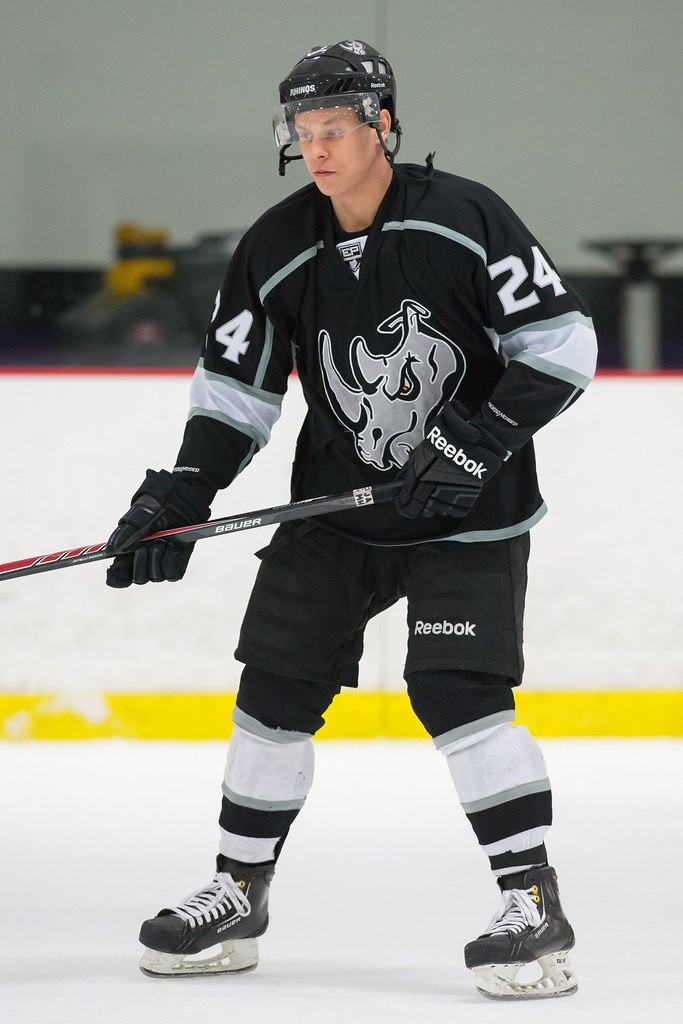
[391,400,505,520]
[105,468,213,589]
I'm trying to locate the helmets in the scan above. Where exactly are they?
[274,39,396,142]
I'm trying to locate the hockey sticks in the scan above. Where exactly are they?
[0,479,406,582]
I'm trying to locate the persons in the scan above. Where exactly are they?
[63,224,192,368]
[105,40,597,999]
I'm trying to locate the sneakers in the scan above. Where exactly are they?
[463,866,577,1002]
[137,855,277,979]
